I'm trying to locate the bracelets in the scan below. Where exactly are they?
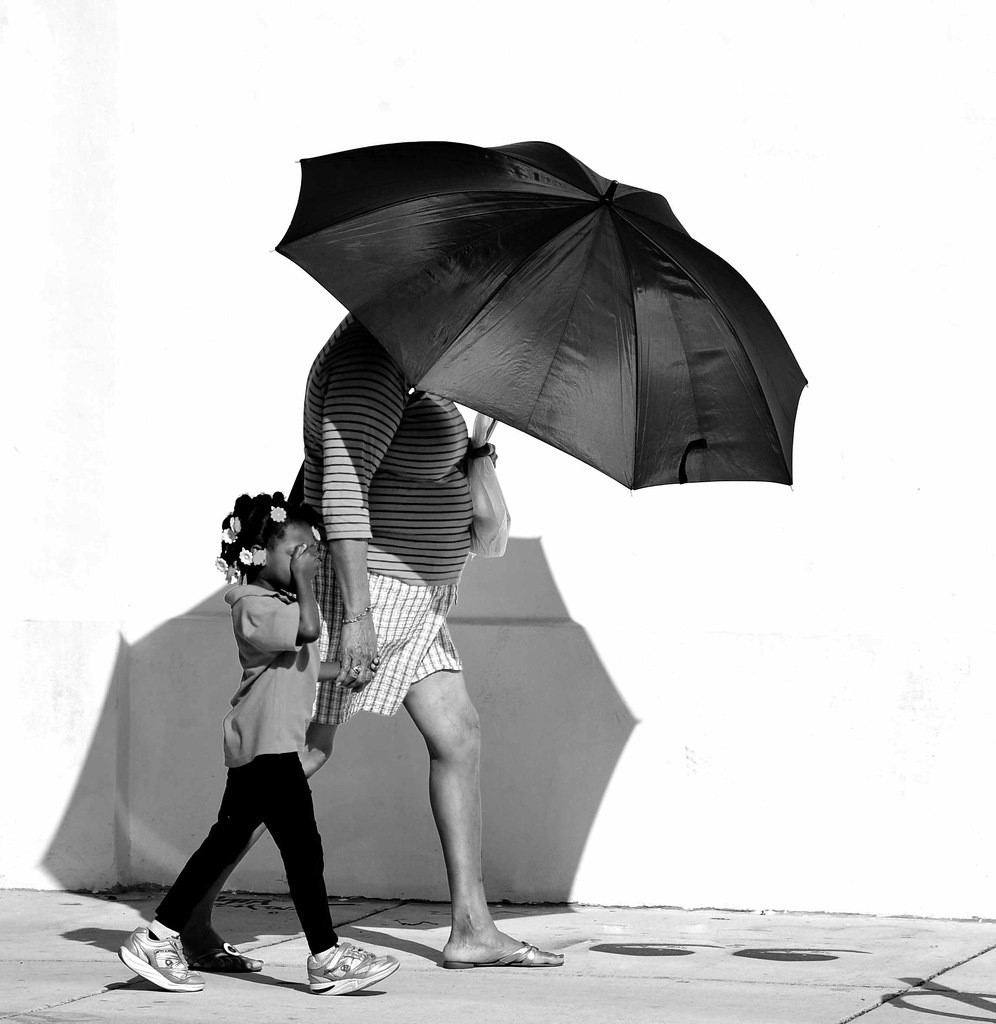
[337,601,376,624]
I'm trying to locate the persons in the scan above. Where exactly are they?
[181,310,564,972]
[118,491,402,997]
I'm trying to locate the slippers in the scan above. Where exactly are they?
[183,942,264,972]
[443,941,564,969]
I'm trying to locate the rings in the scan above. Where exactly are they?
[352,666,360,675]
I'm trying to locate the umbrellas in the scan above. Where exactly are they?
[273,140,808,500]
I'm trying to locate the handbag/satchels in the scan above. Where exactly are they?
[468,413,511,558]
[287,459,305,507]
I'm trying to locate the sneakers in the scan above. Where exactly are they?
[118,926,205,993]
[307,942,400,995]
